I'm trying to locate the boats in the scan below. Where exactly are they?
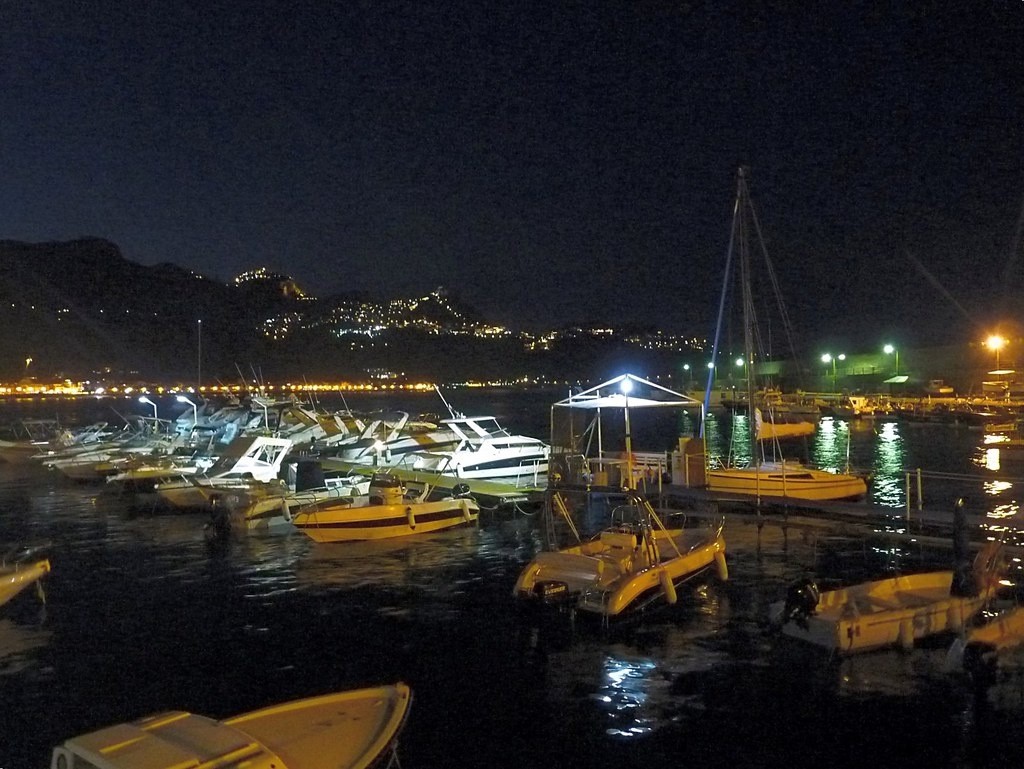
[720,385,1005,423]
[0,372,1023,674]
[52,680,413,769]
[0,557,51,607]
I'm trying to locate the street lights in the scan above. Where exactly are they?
[987,335,1005,382]
[883,344,898,376]
[821,352,846,392]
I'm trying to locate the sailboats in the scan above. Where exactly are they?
[605,162,868,501]
